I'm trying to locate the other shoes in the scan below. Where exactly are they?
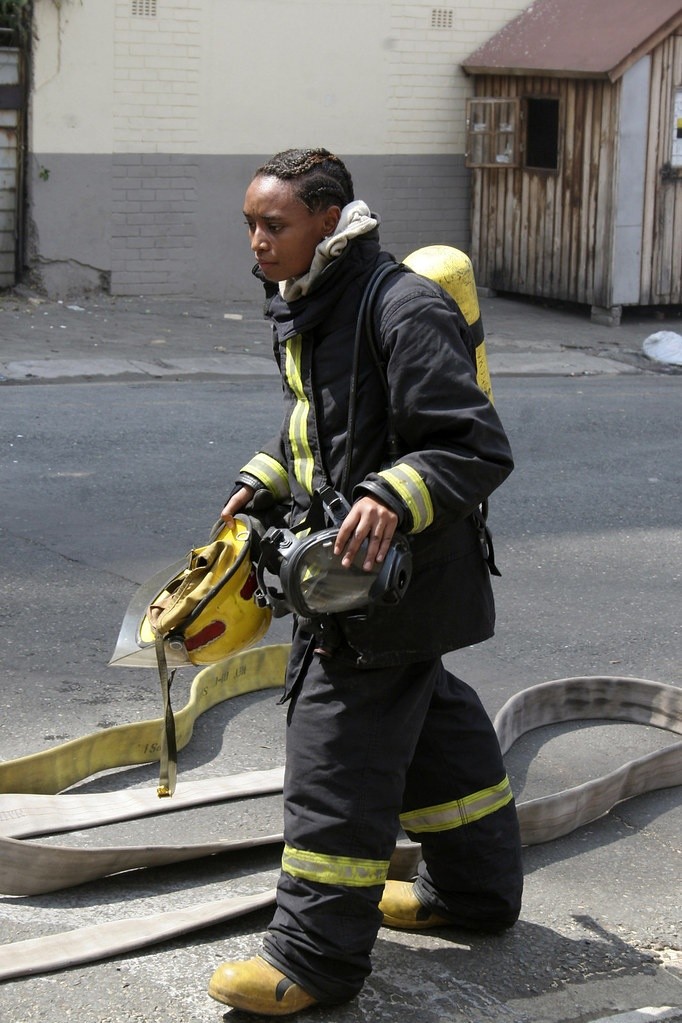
[208,954,316,1015]
[378,880,451,929]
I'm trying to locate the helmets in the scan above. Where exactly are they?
[135,514,273,667]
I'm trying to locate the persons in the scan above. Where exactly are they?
[206,150,516,1013]
